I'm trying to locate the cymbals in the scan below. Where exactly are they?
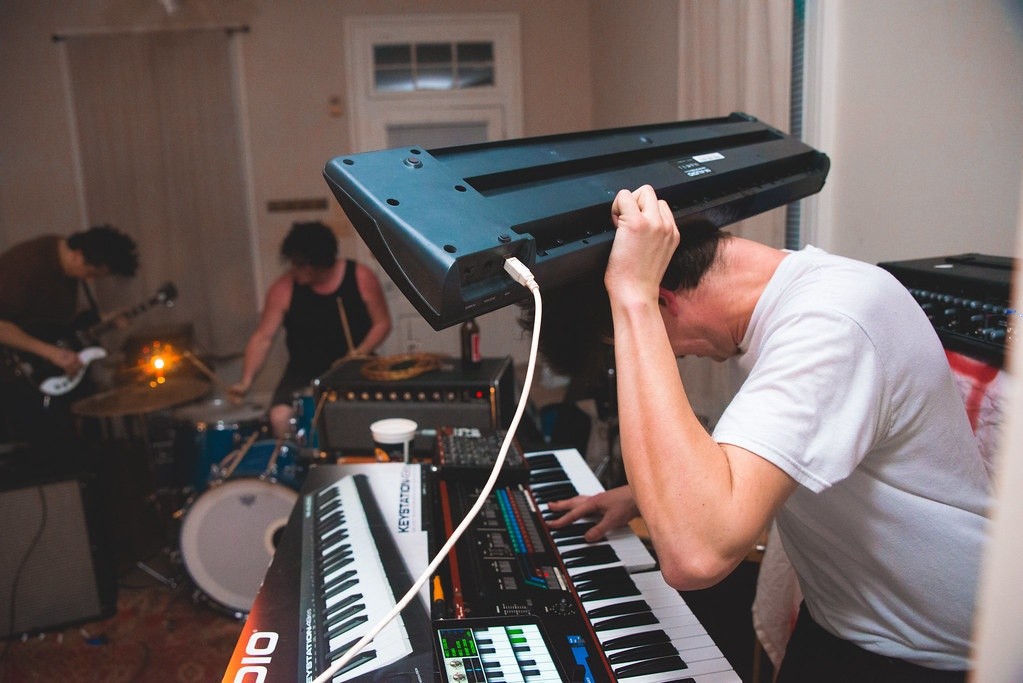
[119,348,204,373]
[74,377,212,418]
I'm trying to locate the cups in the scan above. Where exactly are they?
[369,418,419,463]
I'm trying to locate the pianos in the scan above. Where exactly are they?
[433,443,744,683]
[298,474,433,683]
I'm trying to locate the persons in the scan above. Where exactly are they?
[0,224,143,493]
[228,221,394,443]
[512,222,992,683]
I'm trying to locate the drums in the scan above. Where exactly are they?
[173,395,272,487]
[292,389,323,463]
[177,439,311,621]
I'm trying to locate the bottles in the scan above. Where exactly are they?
[461,318,481,372]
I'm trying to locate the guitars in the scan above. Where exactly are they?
[10,279,180,396]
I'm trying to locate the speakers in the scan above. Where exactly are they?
[315,352,518,457]
[0,438,116,642]
